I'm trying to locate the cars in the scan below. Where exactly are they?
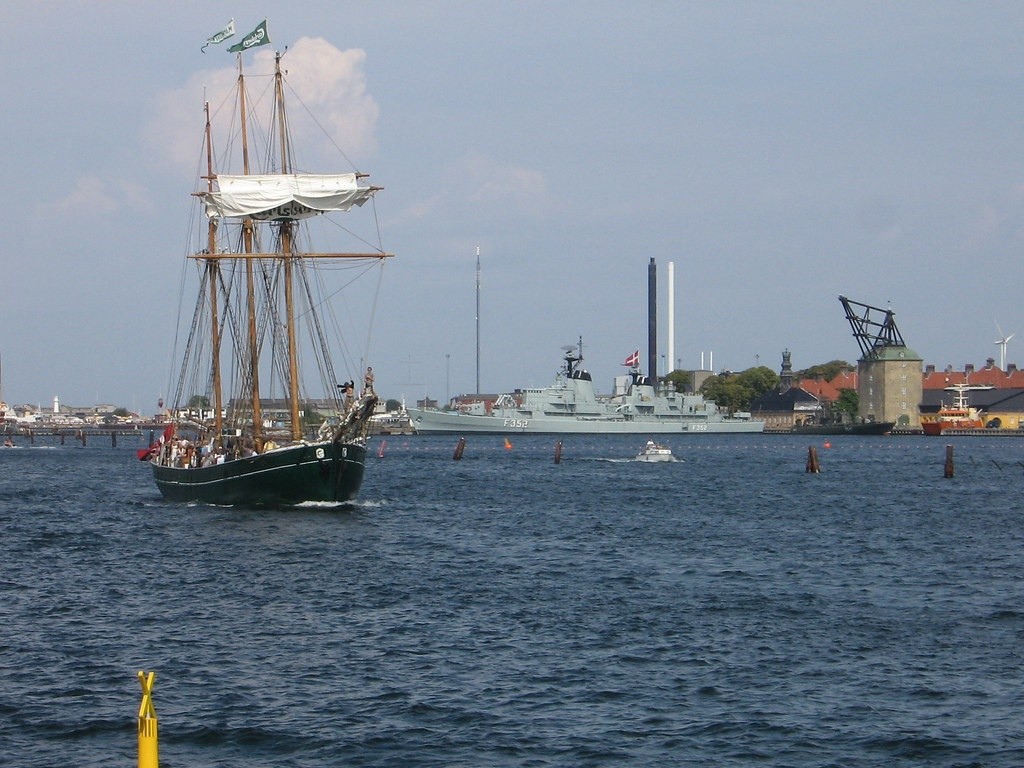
[636,443,673,463]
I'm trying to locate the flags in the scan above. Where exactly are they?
[207,19,235,44]
[230,18,272,54]
[624,349,639,366]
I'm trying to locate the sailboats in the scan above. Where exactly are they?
[140,45,395,507]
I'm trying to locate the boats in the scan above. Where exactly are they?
[789,419,896,434]
[922,382,986,436]
[405,335,766,433]
[2,443,17,448]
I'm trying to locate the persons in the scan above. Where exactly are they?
[364,367,374,394]
[337,380,354,413]
[162,436,278,469]
